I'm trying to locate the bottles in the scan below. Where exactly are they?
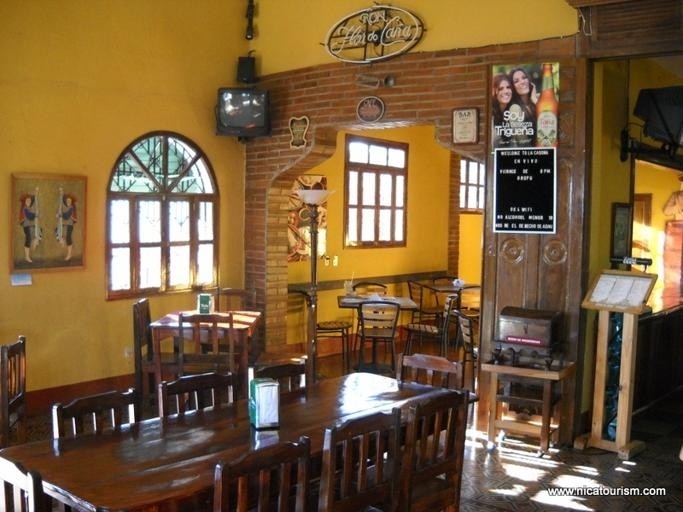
[534,63,559,148]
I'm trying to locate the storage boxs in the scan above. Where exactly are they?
[498,305,565,357]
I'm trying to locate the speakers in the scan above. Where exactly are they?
[237,56,256,83]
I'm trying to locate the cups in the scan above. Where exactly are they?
[344,280,353,297]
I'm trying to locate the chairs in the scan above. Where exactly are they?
[214,287,258,312]
[133,298,186,420]
[314,275,482,380]
[177,313,238,408]
[0,449,43,512]
[212,389,470,512]
[52,358,307,437]
[253,303,317,383]
[397,353,458,389]
[0,335,28,448]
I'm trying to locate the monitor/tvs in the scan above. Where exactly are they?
[215,87,273,138]
[622,85,683,172]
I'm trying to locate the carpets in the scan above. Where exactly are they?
[246,425,683,512]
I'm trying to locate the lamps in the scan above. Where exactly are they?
[297,189,335,295]
[245,0,255,40]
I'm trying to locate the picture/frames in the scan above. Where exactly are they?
[611,202,632,262]
[10,171,88,273]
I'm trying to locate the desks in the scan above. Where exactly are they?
[480,357,576,453]
[148,311,263,400]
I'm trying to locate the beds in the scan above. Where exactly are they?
[0,373,481,512]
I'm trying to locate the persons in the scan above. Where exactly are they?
[490,67,541,152]
[18,192,39,263]
[55,192,78,261]
